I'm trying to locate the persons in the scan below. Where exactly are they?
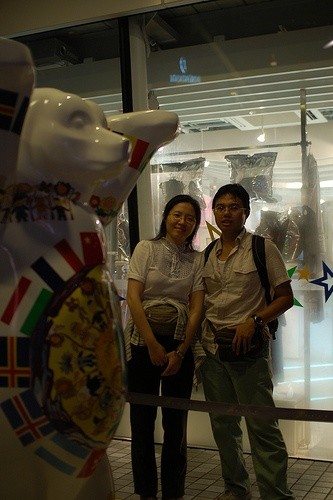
[125,195,206,500]
[197,183,293,500]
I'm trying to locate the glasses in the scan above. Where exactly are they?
[166,212,197,225]
[213,205,245,214]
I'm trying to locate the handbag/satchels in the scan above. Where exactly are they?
[142,306,178,326]
[214,330,263,362]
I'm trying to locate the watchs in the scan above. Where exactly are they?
[251,314,263,329]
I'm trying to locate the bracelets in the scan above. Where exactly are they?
[176,350,184,360]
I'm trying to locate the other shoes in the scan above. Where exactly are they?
[218,492,251,500]
